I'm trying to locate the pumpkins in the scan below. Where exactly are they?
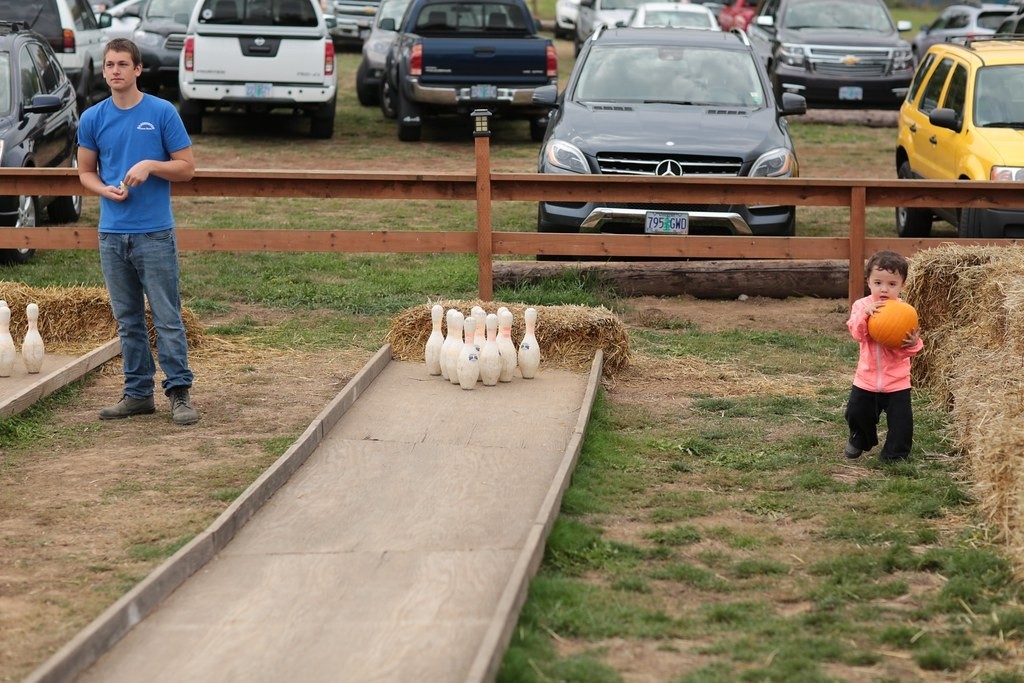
[867,299,918,348]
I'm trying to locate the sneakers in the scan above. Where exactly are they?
[168,387,198,424]
[98,393,155,420]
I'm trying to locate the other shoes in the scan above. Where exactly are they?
[845,440,862,458]
[880,448,908,464]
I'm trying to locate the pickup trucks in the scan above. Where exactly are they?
[379,0,561,144]
[177,0,340,139]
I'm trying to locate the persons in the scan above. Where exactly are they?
[844,250,925,461]
[76,38,197,425]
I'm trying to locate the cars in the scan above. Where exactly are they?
[574,0,669,58]
[126,1,198,95]
[90,1,148,36]
[322,0,382,49]
[616,2,725,67]
[358,0,514,104]
[551,0,585,38]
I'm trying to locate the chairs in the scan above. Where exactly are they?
[428,11,447,25]
[489,13,507,26]
[215,0,238,20]
[278,2,303,21]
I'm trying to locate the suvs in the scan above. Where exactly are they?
[911,0,1022,65]
[993,6,1024,42]
[530,24,807,260]
[0,18,80,262]
[0,0,115,115]
[744,0,918,107]
[894,31,1024,240]
